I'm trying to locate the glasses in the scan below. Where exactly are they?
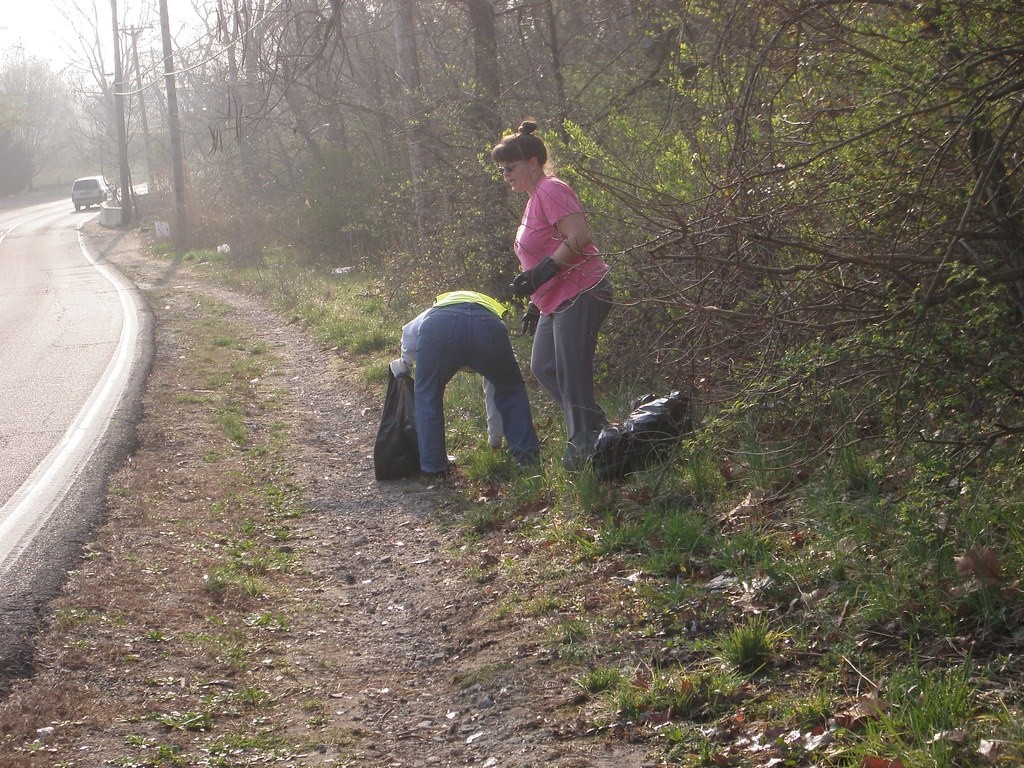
[497,161,525,175]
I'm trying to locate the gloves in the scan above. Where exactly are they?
[520,302,541,335]
[509,256,561,298]
[389,358,408,378]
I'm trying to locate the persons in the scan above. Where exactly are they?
[492,121,614,475]
[389,291,540,483]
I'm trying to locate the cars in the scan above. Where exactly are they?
[72,175,116,211]
